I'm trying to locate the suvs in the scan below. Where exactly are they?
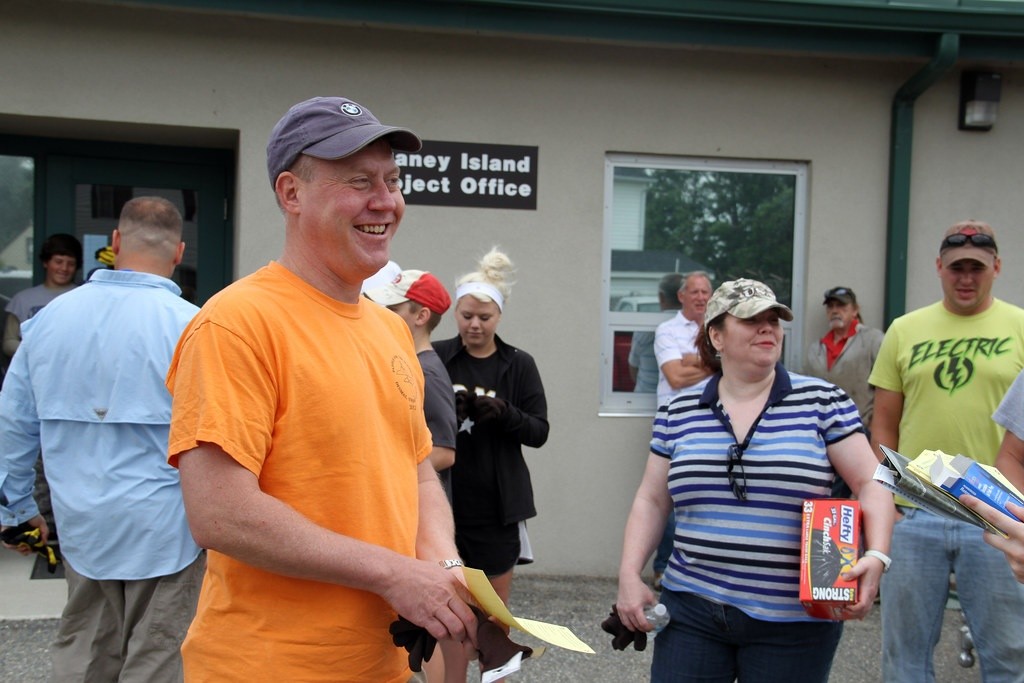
[0,266,34,298]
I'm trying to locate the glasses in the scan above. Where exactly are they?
[940,233,997,254]
[725,444,748,503]
[824,290,852,298]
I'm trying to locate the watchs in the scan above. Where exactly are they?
[864,550,893,573]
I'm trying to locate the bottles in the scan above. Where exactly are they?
[639,603,672,644]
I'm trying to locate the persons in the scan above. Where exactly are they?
[654,272,712,409]
[362,263,457,504]
[166,94,510,683]
[0,196,208,683]
[868,219,1024,683]
[628,273,685,394]
[0,233,84,513]
[805,287,885,604]
[429,249,548,683]
[616,277,896,683]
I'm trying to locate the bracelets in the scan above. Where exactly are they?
[439,559,463,568]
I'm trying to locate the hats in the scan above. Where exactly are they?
[703,277,794,345]
[823,287,856,306]
[364,269,452,315]
[266,96,423,192]
[361,260,402,294]
[939,218,997,267]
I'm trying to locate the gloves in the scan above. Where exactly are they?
[389,604,534,674]
[0,519,62,575]
[470,395,508,426]
[455,389,475,423]
[601,603,647,651]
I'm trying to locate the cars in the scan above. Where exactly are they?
[614,297,662,314]
